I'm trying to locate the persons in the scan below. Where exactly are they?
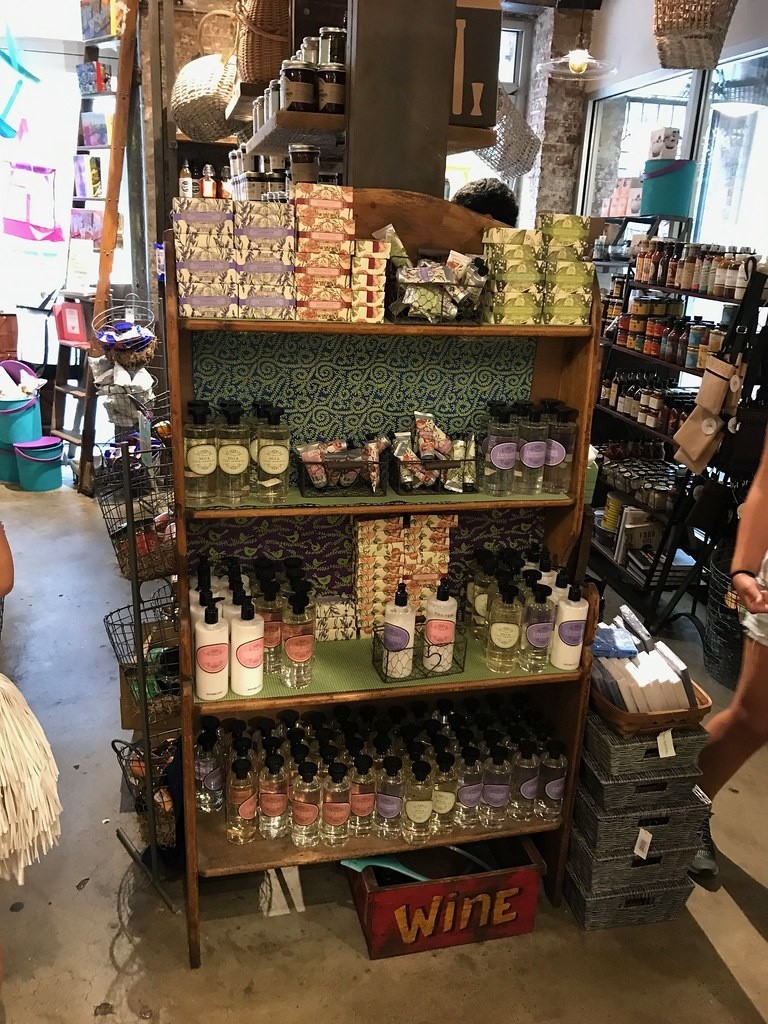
[690,427,768,878]
[0,521,15,632]
[452,178,518,227]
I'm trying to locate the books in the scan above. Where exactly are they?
[613,507,697,586]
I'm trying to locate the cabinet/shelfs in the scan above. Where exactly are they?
[587,214,766,626]
[160,189,600,969]
[248,0,494,199]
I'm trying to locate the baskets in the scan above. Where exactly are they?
[387,265,486,325]
[171,0,290,142]
[297,455,388,498]
[89,305,183,855]
[654,0,738,71]
[370,625,467,682]
[388,451,480,496]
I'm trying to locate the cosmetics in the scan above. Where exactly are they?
[393,411,476,493]
[385,583,415,678]
[467,543,588,673]
[297,430,396,492]
[195,697,568,845]
[189,555,316,700]
[183,397,290,507]
[372,224,489,323]
[422,577,456,671]
[479,400,578,497]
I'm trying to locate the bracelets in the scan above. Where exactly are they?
[731,570,755,579]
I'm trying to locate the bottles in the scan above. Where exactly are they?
[130,644,179,700]
[599,366,697,436]
[253,562,316,690]
[423,578,458,674]
[381,583,415,680]
[634,239,768,301]
[183,397,291,506]
[464,545,556,675]
[193,692,567,846]
[177,156,231,199]
[476,396,579,498]
[658,319,690,367]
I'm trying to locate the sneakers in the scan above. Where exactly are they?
[688,811,721,876]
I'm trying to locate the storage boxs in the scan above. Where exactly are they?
[344,834,547,959]
[315,513,458,642]
[176,183,596,326]
[449,7,502,126]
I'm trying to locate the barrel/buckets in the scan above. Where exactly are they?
[0,360,64,493]
[600,491,634,532]
[640,158,697,218]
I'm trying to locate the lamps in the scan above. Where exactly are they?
[537,10,615,82]
